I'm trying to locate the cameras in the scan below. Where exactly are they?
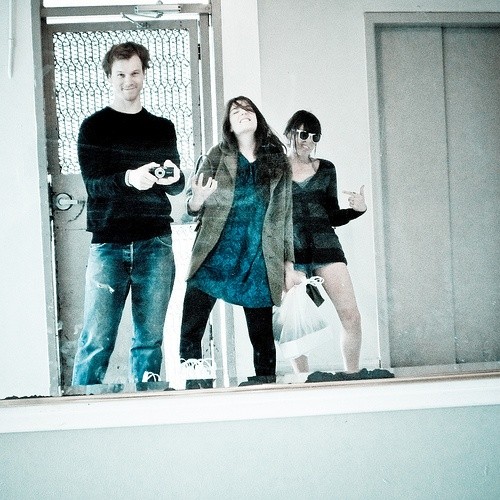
[149,166,175,179]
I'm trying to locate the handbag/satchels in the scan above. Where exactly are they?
[270,275,345,371]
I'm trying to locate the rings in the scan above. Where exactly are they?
[352,192,356,196]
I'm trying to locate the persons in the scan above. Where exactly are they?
[70,42,186,385]
[178,94,296,379]
[282,110,367,373]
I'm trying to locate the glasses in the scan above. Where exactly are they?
[291,128,321,144]
